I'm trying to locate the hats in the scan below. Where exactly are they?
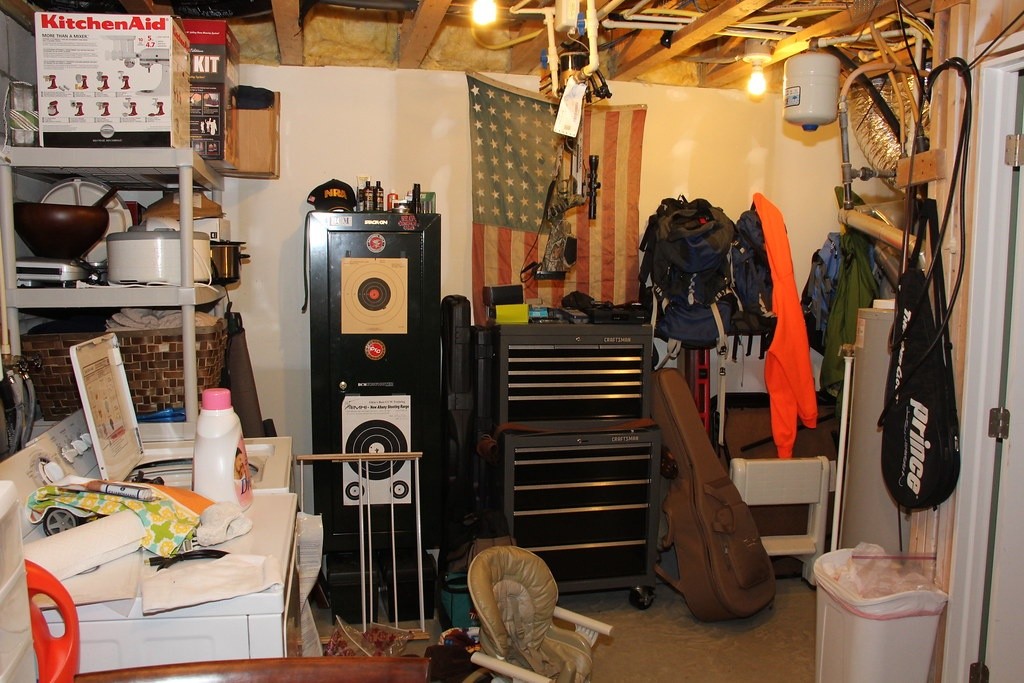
[307,179,357,213]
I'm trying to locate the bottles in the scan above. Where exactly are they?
[387,189,398,212]
[363,181,374,212]
[374,181,384,213]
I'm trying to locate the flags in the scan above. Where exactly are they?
[465,68,648,327]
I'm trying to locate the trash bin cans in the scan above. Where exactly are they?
[813,545,950,683]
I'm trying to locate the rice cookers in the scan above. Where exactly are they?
[106,225,212,284]
[210,240,250,283]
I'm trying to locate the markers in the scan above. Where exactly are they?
[86,478,152,503]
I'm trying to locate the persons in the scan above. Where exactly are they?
[234,447,245,479]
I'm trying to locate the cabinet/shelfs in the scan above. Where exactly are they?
[0,143,222,445]
[485,322,663,611]
[310,213,443,600]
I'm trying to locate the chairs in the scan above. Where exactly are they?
[468,546,616,683]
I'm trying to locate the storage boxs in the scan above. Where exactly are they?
[189,86,241,171]
[35,13,190,150]
[181,18,242,86]
[326,553,379,625]
[224,92,283,180]
[380,553,437,623]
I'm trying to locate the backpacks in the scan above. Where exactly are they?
[801,231,881,356]
[637,195,777,351]
